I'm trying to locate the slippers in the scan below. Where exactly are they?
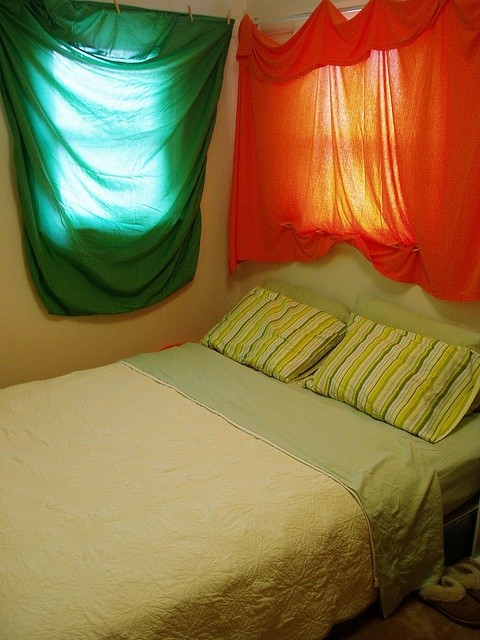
[419,576,480,629]
[445,562,480,603]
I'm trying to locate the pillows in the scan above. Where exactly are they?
[299,311,480,442]
[260,276,351,329]
[200,285,345,382]
[354,291,480,417]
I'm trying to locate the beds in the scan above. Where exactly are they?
[0,280,480,640]
